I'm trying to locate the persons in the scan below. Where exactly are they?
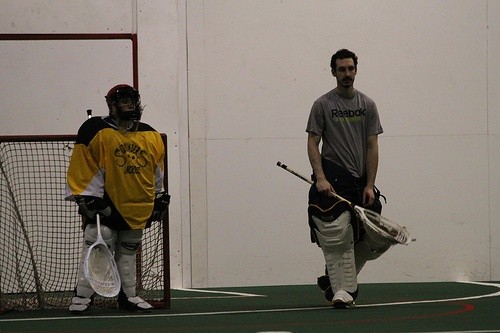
[65,84,167,315]
[306,49,384,308]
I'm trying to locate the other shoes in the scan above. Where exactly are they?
[69,296,92,312]
[331,290,355,307]
[118,296,153,310]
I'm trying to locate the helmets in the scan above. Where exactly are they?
[105,83,141,120]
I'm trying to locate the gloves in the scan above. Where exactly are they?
[151,192,171,222]
[74,196,112,219]
